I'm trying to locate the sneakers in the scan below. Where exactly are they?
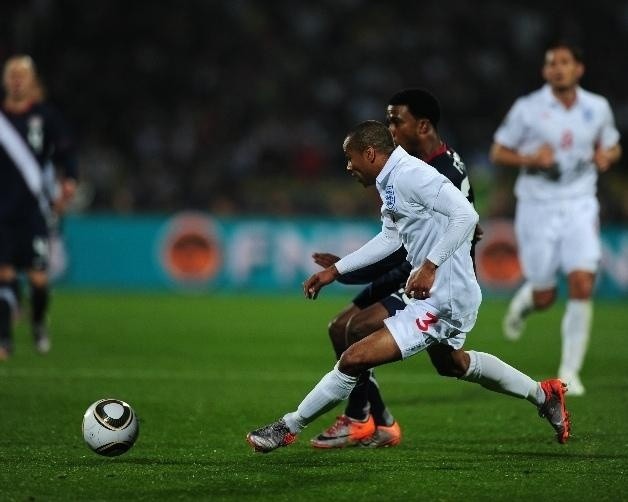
[558,365,585,395]
[503,289,525,340]
[311,414,375,448]
[354,421,401,449]
[247,419,296,450]
[32,321,51,353]
[540,379,571,443]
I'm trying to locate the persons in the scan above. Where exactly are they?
[0,51,81,351]
[488,43,622,397]
[308,87,484,453]
[0,114,59,367]
[242,118,575,455]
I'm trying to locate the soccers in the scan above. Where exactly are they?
[81,399,139,456]
